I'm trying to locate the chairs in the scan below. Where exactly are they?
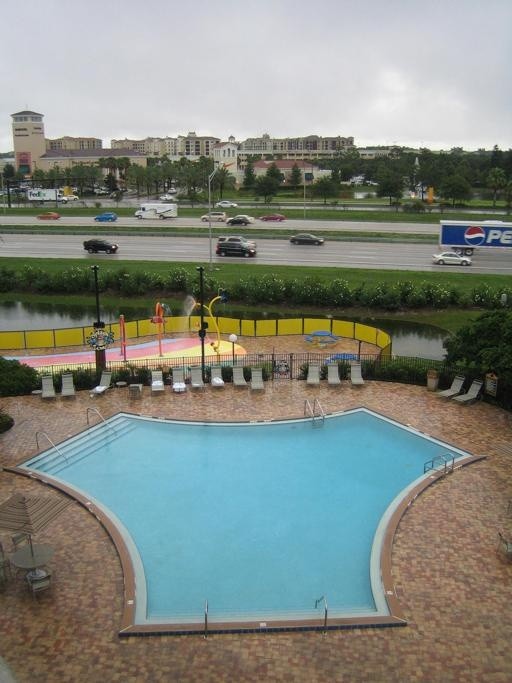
[438,375,484,404]
[41,364,265,401]
[0,542,13,583]
[12,532,30,547]
[30,574,51,598]
[306,362,365,388]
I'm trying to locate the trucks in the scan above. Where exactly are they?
[133,202,178,220]
[438,218,511,257]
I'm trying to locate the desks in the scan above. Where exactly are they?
[13,545,52,581]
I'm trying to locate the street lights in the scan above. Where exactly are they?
[196,264,209,383]
[207,161,226,269]
[227,331,238,363]
[89,263,108,387]
[31,159,61,173]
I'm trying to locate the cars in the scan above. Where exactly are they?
[215,200,237,208]
[290,231,325,246]
[0,182,109,203]
[215,235,257,257]
[37,211,62,221]
[430,251,473,268]
[82,238,119,253]
[94,210,118,224]
[202,210,285,227]
[158,180,176,201]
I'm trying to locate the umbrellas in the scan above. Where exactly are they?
[0,492,77,556]
